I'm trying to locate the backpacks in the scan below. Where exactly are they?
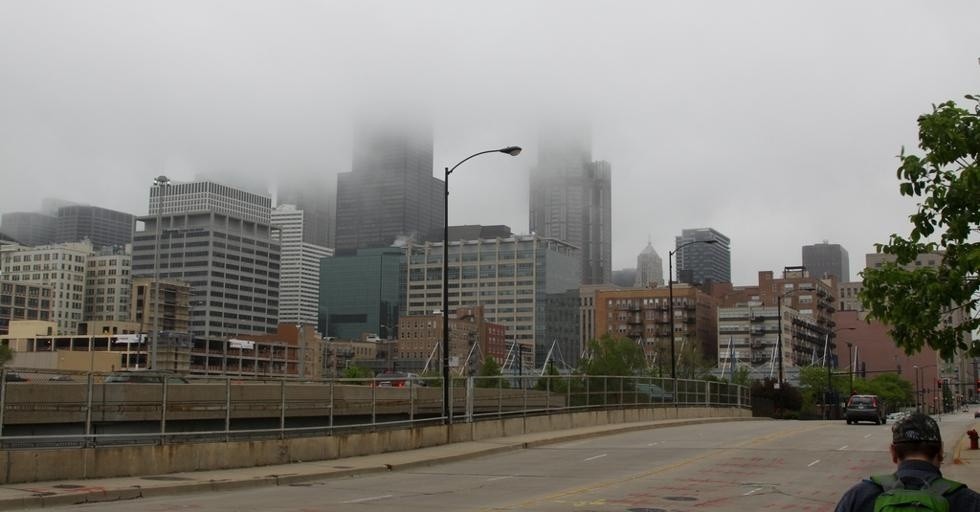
[872,475,965,512]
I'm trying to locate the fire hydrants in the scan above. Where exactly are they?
[967,428,980,450]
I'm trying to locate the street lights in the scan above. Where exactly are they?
[668,239,714,382]
[150,173,171,377]
[777,286,816,386]
[441,145,522,425]
[828,327,856,395]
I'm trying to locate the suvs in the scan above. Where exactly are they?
[844,393,888,426]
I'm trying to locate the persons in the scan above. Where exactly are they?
[835,413,980,511]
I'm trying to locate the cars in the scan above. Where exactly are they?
[1,368,192,385]
[369,372,429,389]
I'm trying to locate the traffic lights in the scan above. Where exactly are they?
[938,380,941,388]
[976,379,980,392]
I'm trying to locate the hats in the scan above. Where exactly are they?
[892,414,940,442]
